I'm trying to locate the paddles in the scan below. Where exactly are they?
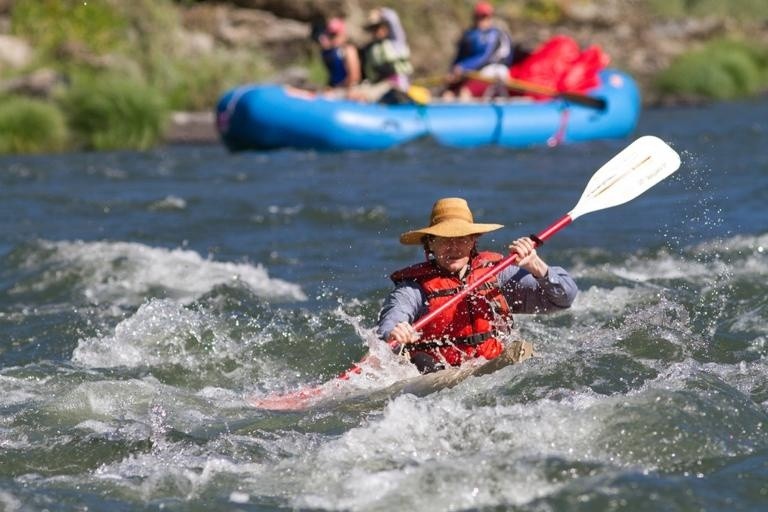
[255,135,682,410]
[457,71,606,112]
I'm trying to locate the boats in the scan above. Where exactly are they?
[243,339,535,413]
[214,61,647,152]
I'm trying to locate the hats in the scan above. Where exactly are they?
[472,2,494,17]
[400,196,505,246]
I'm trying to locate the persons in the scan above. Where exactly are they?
[348,5,414,105]
[376,196,577,373]
[284,16,362,100]
[440,1,513,104]
